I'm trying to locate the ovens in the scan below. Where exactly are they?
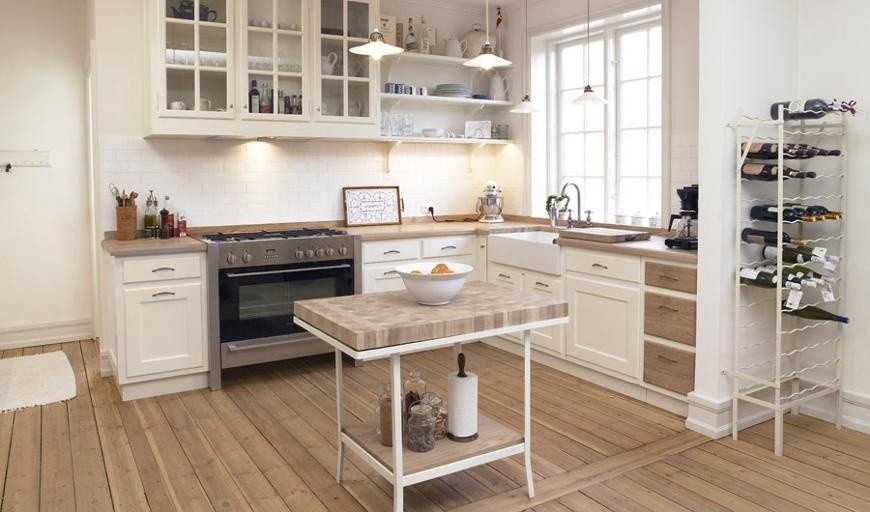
[207,259,361,370]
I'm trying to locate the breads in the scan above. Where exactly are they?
[431,264,453,273]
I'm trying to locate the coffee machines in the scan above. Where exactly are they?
[664,184,698,250]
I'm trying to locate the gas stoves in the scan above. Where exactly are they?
[201,225,354,268]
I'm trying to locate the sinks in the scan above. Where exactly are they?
[488,231,561,276]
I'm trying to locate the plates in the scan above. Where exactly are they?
[432,84,470,97]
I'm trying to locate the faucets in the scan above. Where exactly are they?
[560,181,581,226]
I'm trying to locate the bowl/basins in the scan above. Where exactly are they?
[423,129,445,137]
[394,261,474,305]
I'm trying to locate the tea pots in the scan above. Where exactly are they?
[441,35,468,58]
[170,0,218,22]
[488,72,511,100]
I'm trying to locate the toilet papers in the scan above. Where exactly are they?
[446,373,479,439]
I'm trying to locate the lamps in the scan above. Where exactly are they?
[508,0,544,114]
[463,0,512,71]
[571,1,608,105]
[349,0,404,60]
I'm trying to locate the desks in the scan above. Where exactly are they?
[292,280,570,512]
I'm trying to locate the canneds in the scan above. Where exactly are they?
[385,83,427,95]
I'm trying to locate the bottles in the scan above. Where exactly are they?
[405,15,430,53]
[144,189,187,240]
[249,79,302,115]
[380,371,448,453]
[740,97,857,325]
[492,123,499,139]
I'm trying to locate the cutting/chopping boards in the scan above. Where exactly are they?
[559,227,650,244]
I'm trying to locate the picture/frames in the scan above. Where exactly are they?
[343,186,402,227]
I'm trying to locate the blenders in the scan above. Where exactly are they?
[476,183,505,224]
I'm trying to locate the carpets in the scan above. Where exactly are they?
[0,349,77,414]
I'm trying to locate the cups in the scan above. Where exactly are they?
[321,52,338,74]
[170,101,186,110]
[348,99,362,117]
[381,112,414,137]
[199,97,212,111]
[116,207,137,241]
[406,84,428,96]
[498,124,509,139]
[249,19,301,31]
[349,59,362,76]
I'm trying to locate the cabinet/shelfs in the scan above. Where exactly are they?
[487,261,566,364]
[102,231,210,404]
[725,105,848,457]
[376,52,516,145]
[563,244,641,387]
[642,254,697,404]
[143,0,379,142]
[353,230,480,366]
